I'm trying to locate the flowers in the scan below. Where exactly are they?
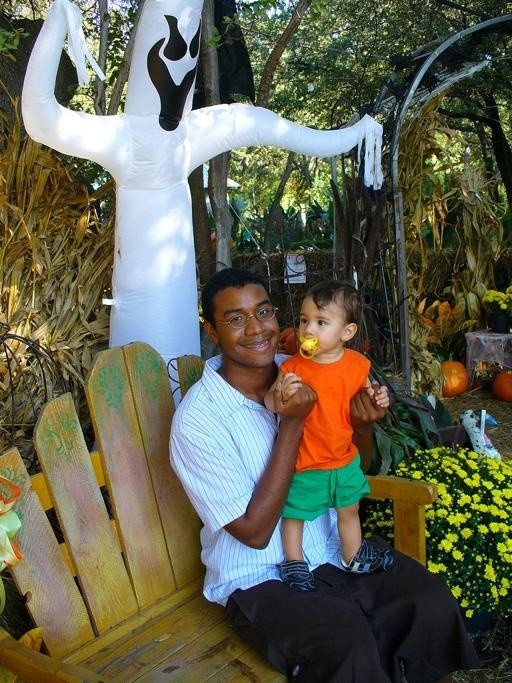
[363,444,512,619]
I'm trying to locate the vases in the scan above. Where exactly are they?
[460,606,494,631]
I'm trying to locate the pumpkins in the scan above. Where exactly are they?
[277,326,298,353]
[442,352,469,398]
[210,230,235,253]
[492,369,512,401]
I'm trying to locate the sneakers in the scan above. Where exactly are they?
[276,562,315,592]
[341,540,398,573]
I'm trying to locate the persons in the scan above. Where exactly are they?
[168,267,483,682]
[262,279,399,593]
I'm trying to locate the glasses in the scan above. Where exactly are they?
[216,307,278,328]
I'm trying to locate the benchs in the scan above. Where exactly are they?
[0,342,438,683]
